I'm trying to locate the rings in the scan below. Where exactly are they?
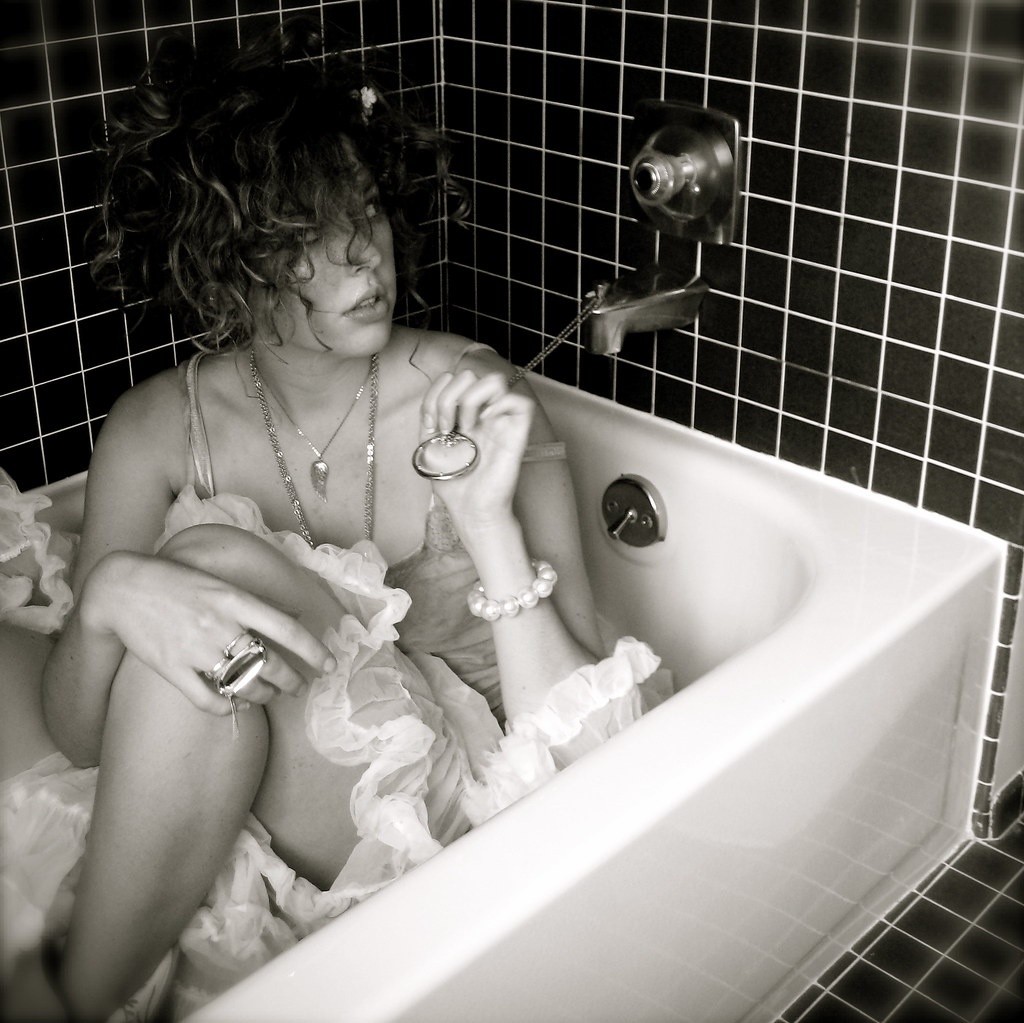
[205,629,268,738]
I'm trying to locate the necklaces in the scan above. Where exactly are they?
[249,347,380,550]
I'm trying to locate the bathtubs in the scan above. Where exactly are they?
[0,364,997,1018]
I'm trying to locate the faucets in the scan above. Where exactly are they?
[585,266,710,354]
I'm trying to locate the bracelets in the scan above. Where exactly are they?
[468,558,557,622]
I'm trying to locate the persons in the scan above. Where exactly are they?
[42,30,608,1022]
